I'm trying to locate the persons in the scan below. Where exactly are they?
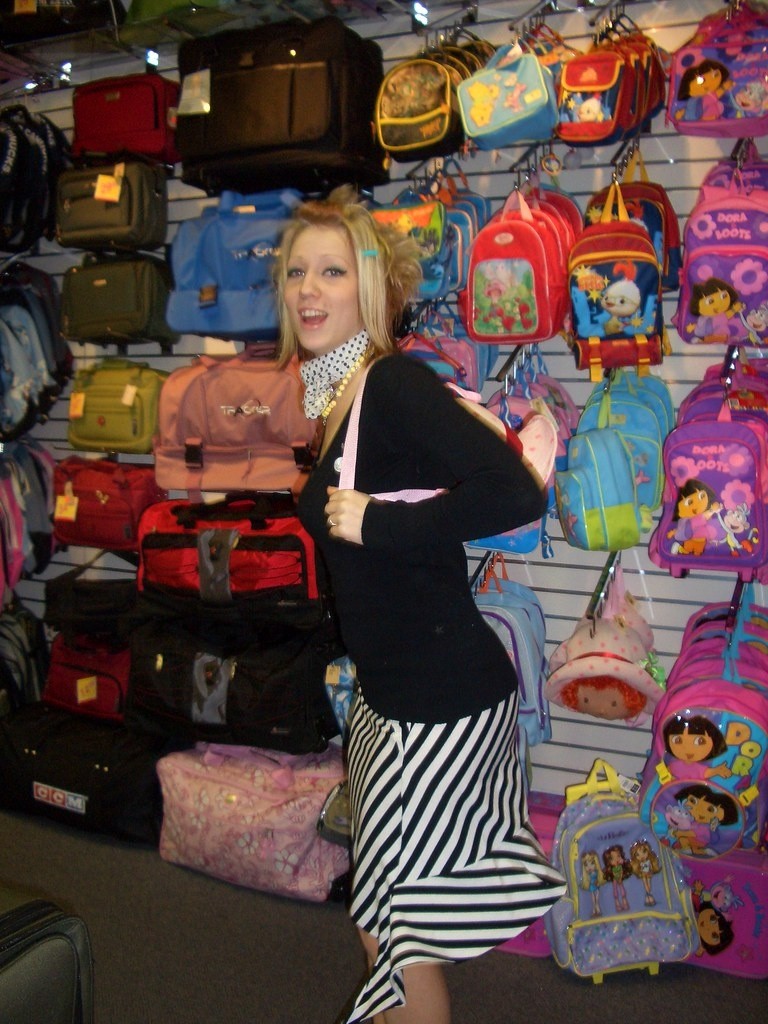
[272,200,567,1023]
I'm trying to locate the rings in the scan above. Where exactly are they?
[329,520,336,526]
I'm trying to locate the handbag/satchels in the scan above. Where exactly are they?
[0,701,351,906]
[33,22,391,752]
[338,362,557,507]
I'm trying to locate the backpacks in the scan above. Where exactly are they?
[0,104,77,767]
[369,0,768,985]
